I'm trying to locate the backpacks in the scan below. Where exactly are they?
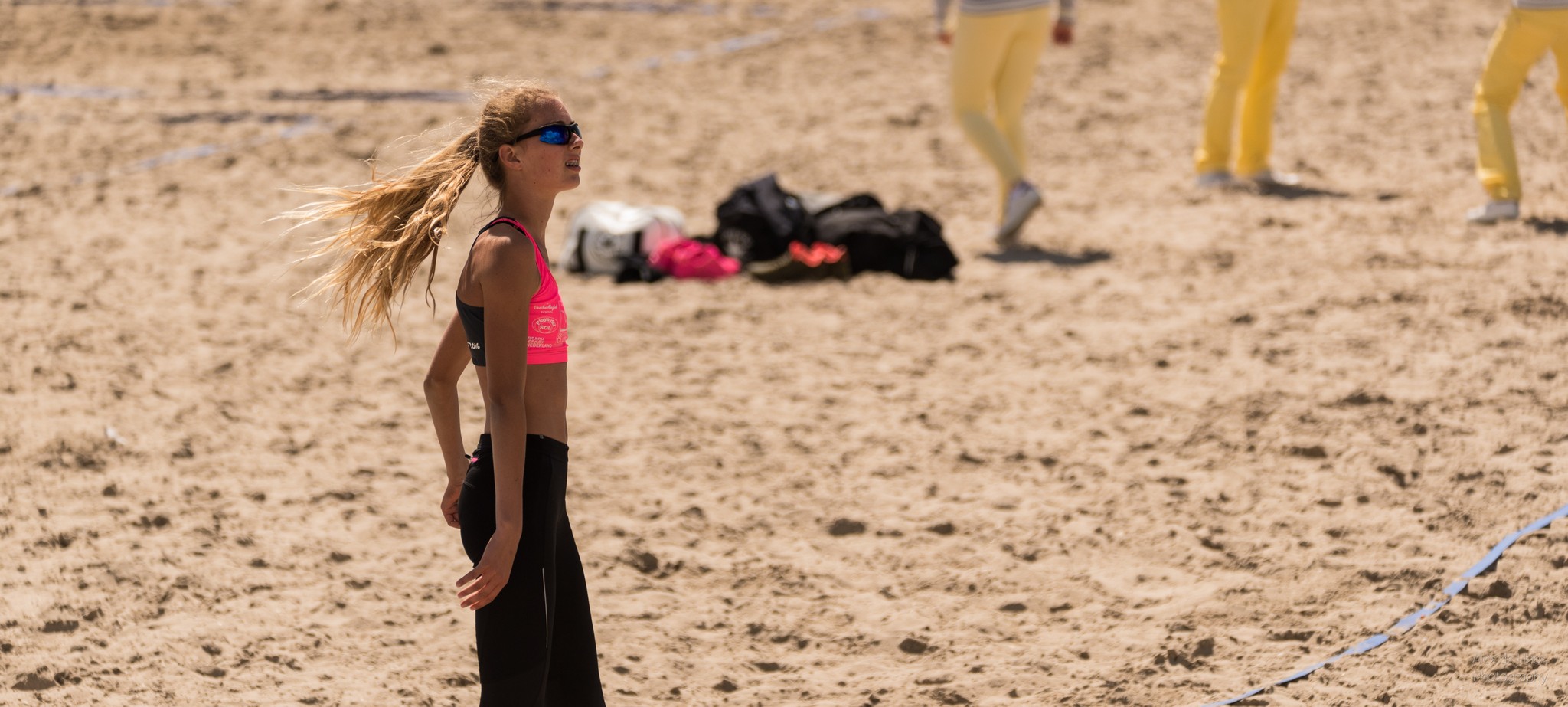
[751,191,960,285]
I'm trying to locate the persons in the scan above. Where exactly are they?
[1467,0,1568,224]
[273,82,607,707]
[1193,0,1308,197]
[934,0,1075,257]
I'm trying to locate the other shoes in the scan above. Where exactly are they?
[1467,199,1518,221]
[995,185,1042,246]
[1198,167,1302,194]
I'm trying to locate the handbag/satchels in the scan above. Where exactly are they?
[564,201,687,283]
[698,173,816,261]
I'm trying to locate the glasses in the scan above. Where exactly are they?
[504,120,585,145]
